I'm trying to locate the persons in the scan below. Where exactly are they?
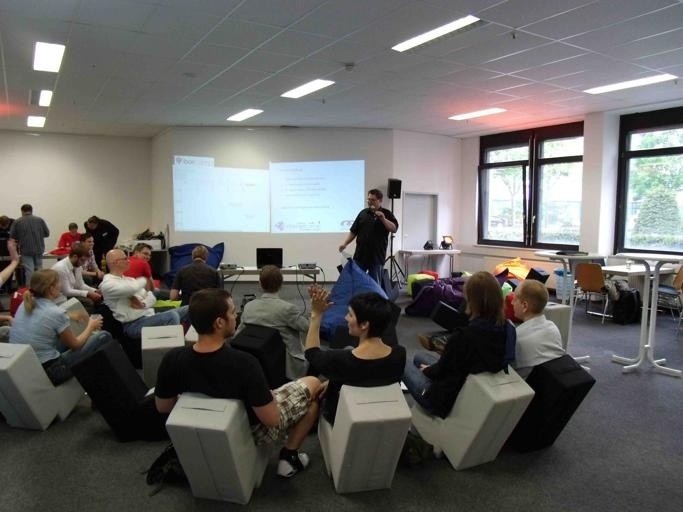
[403,271,516,419]
[48,246,102,315]
[232,264,324,383]
[79,233,105,288]
[58,223,81,250]
[9,269,122,388]
[510,279,565,383]
[304,289,406,435]
[339,189,398,287]
[98,248,190,338]
[122,243,159,299]
[84,215,119,271]
[1,216,26,295]
[155,287,322,478]
[169,244,219,305]
[8,206,49,289]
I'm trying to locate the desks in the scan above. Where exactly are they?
[399,247,461,287]
[533,249,605,374]
[601,263,674,312]
[611,252,682,376]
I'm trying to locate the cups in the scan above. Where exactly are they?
[92,314,102,334]
[625,261,632,269]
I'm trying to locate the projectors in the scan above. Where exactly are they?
[298,263,316,269]
[220,263,237,269]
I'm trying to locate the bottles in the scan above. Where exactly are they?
[341,250,352,260]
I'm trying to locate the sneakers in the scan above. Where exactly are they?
[277,452,309,478]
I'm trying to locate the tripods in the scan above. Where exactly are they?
[384,198,408,289]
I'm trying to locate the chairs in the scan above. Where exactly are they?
[565,255,605,319]
[568,262,612,324]
[641,265,683,322]
[0,266,596,512]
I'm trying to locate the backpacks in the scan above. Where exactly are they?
[147,442,190,488]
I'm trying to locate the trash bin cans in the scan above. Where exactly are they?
[554,268,572,301]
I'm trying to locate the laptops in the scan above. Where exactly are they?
[256,247,283,268]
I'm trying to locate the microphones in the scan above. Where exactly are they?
[371,205,381,217]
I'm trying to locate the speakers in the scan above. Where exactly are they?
[387,178,401,198]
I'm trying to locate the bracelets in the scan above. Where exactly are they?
[96,272,98,277]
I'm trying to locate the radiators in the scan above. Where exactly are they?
[626,273,673,306]
